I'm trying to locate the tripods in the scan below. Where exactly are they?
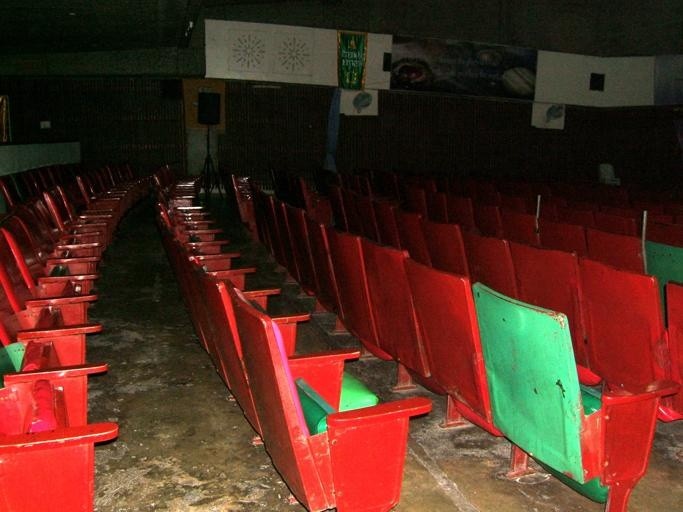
[200,125,223,202]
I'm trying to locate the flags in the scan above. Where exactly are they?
[202,18,395,116]
[530,49,657,131]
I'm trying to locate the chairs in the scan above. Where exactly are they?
[0,162,682,512]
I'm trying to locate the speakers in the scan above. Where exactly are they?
[197,91,220,125]
